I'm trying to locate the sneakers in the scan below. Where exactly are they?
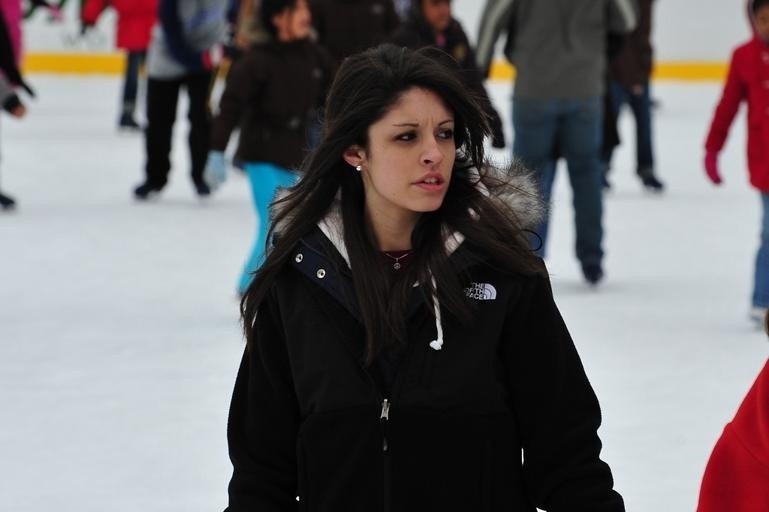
[135,175,167,195]
[582,264,603,283]
[121,115,140,126]
[644,175,662,188]
[192,175,210,193]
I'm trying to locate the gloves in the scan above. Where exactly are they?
[705,151,721,183]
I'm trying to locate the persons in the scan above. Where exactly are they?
[474,1,664,282]
[706,2,768,320]
[221,44,625,512]
[0,0,34,205]
[79,2,505,305]
